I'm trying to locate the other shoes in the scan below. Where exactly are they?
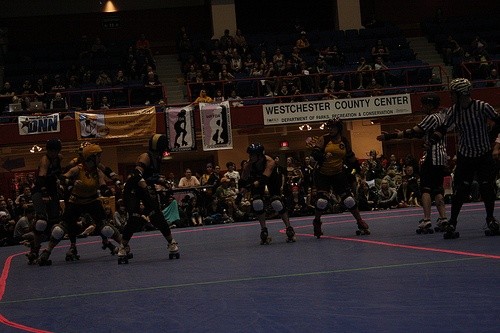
[77,233,88,238]
[199,223,203,226]
[194,224,198,227]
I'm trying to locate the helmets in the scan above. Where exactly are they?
[149,134,168,154]
[328,117,343,134]
[47,140,61,151]
[247,143,264,153]
[421,95,440,107]
[450,78,472,96]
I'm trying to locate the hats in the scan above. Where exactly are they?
[82,143,102,160]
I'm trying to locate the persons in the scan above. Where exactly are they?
[436,32,500,87]
[178,24,400,107]
[74,178,159,239]
[273,155,346,218]
[0,172,37,246]
[116,134,180,265]
[351,150,422,211]
[37,141,122,267]
[310,117,371,238]
[241,142,296,245]
[445,155,481,202]
[65,143,119,262]
[156,160,257,228]
[0,34,162,113]
[26,139,66,267]
[423,78,500,240]
[377,93,455,235]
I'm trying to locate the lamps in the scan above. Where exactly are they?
[299,124,305,131]
[320,124,325,129]
[371,119,379,125]
[306,124,312,130]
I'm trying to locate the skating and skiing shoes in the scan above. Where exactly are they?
[117,247,128,264]
[416,219,433,234]
[29,251,39,265]
[260,227,272,245]
[356,220,370,235]
[168,239,179,259]
[64,246,80,262]
[38,250,52,266]
[483,216,500,236]
[434,217,449,232]
[444,220,459,239]
[313,219,323,239]
[285,226,296,243]
